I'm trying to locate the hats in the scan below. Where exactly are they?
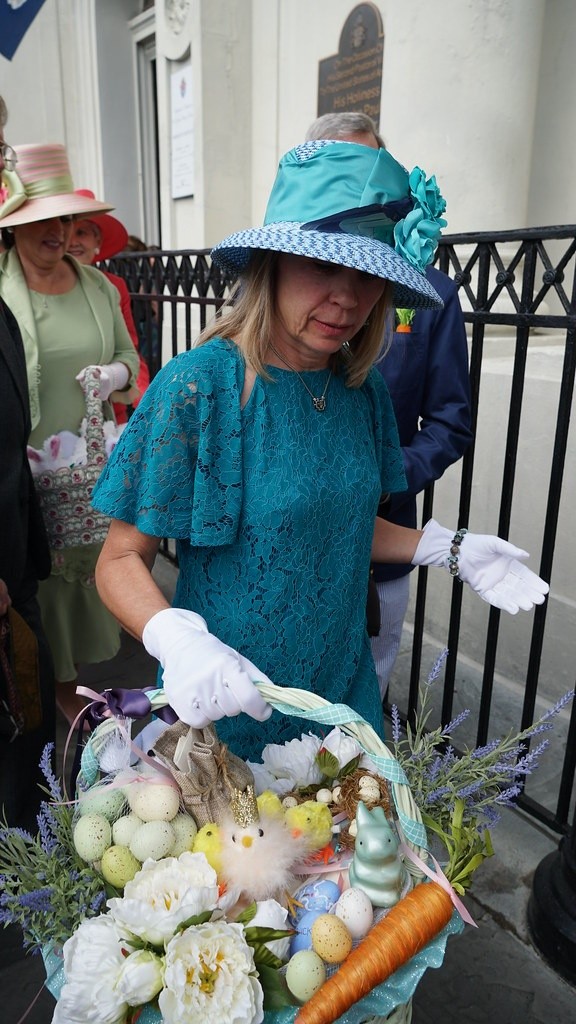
[209,140,445,313]
[0,143,115,226]
[64,188,129,265]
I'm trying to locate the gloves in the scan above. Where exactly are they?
[75,361,130,401]
[410,518,550,615]
[142,607,274,730]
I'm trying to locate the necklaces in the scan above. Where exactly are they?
[267,341,332,412]
[32,285,51,308]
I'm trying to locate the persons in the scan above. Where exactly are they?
[307,112,478,702]
[0,97,163,895]
[84,140,548,762]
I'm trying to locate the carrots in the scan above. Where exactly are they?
[292,882,455,1024]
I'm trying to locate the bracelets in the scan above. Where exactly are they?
[106,364,119,390]
[446,529,468,582]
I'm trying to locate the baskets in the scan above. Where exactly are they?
[32,368,116,589]
[36,682,430,1024]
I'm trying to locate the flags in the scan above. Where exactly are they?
[0,0,46,62]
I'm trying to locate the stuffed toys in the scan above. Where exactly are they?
[192,790,333,917]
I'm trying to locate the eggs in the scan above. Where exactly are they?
[73,770,196,889]
[286,886,374,1003]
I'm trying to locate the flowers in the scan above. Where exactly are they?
[0,651,576,1024]
